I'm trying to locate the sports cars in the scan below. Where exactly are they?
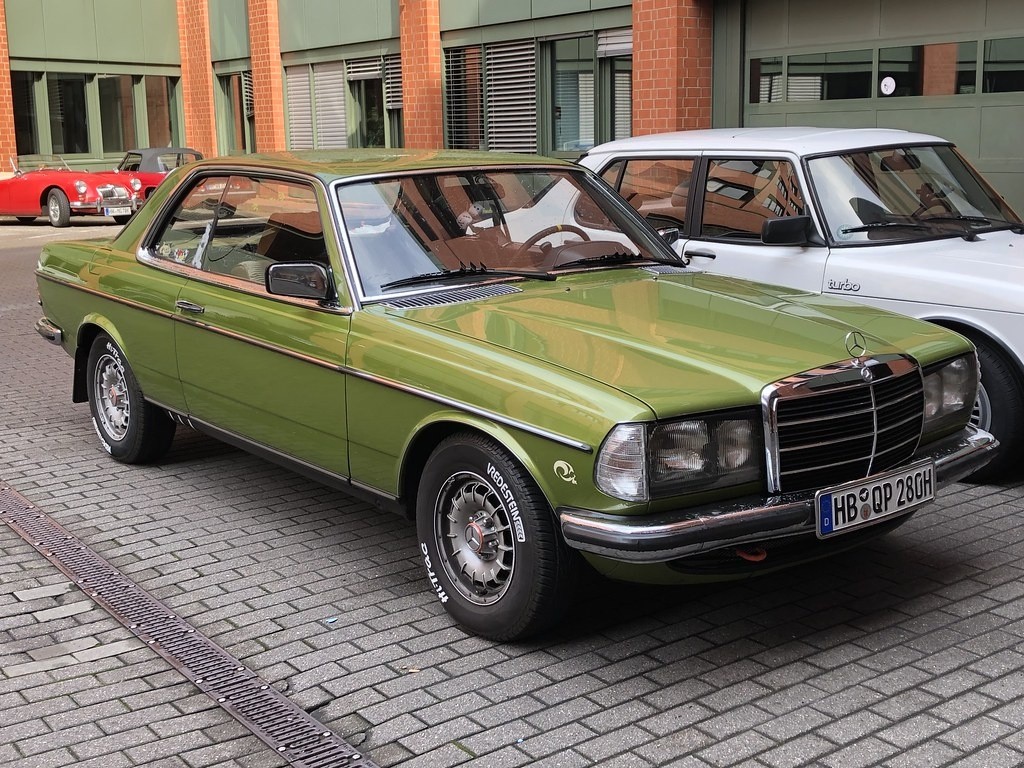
[0,153,146,228]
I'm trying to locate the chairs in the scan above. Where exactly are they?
[620,179,690,221]
[231,210,328,284]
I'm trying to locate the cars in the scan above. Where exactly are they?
[112,148,205,201]
[461,126,1024,488]
[34,146,1003,645]
